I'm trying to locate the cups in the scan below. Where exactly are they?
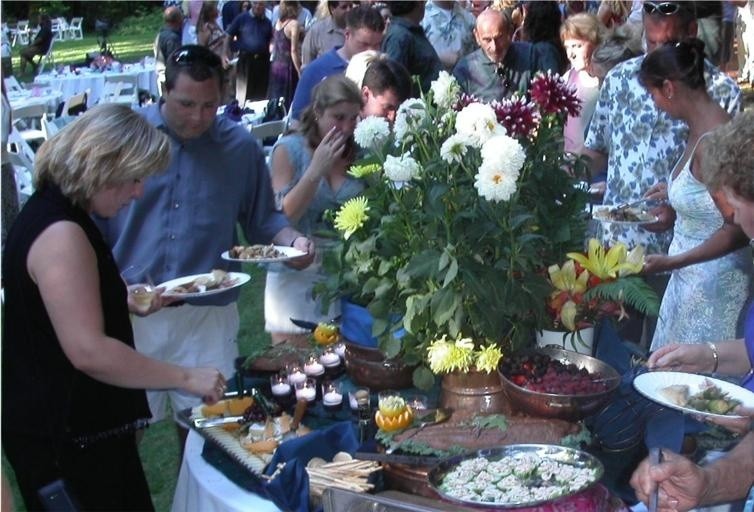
[267,342,430,419]
[118,262,156,306]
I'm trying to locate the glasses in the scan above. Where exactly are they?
[174,50,221,68]
[644,2,680,15]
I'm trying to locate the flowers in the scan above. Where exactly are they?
[323,67,661,379]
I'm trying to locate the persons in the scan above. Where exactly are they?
[16,14,52,77]
[0,102,228,512]
[0,13,14,77]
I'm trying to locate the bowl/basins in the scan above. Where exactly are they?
[426,442,605,510]
[497,342,622,427]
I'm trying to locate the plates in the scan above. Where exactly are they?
[220,245,311,262]
[157,271,251,299]
[629,370,754,422]
[587,201,660,226]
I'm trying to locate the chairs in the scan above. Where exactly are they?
[1,13,289,214]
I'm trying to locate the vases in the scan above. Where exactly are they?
[438,359,511,420]
